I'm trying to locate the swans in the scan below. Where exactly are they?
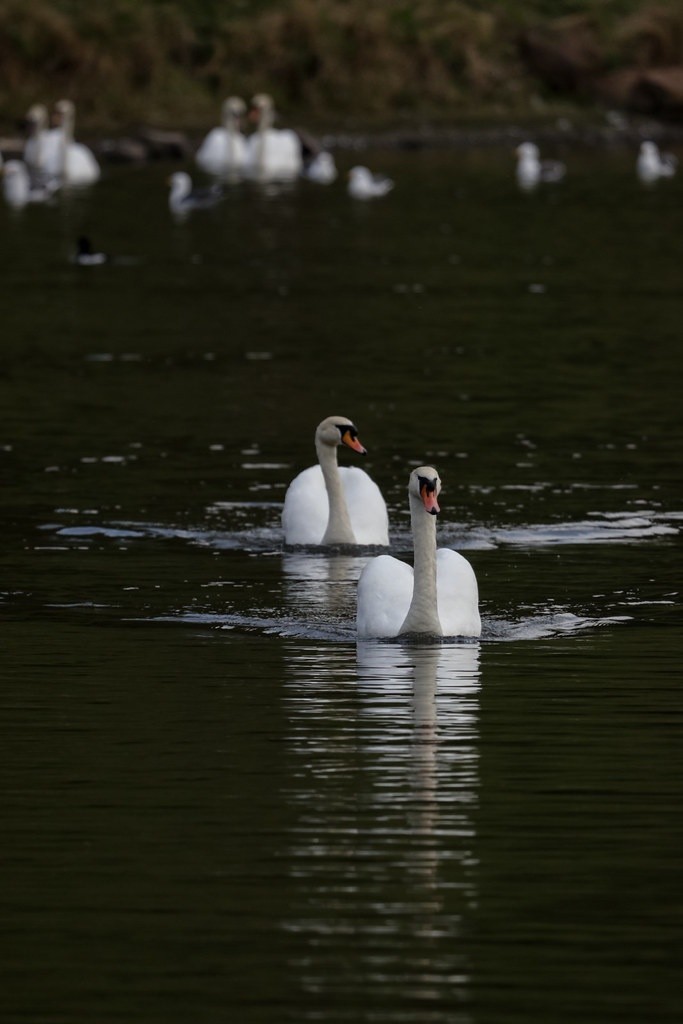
[2,92,677,216]
[281,413,484,642]
